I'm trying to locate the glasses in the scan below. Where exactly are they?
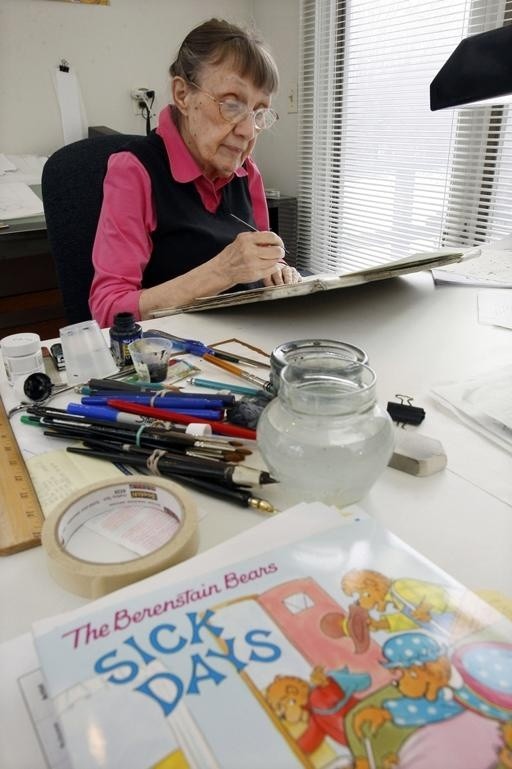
[186,79,280,131]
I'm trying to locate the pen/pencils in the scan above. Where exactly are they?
[229,214,291,255]
[21,351,281,514]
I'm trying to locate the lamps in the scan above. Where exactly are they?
[430,22,512,109]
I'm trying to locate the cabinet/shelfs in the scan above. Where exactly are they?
[0,183,61,298]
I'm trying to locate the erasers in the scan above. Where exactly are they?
[389,427,447,476]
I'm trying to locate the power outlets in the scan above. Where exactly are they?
[134,90,157,115]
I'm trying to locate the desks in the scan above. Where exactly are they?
[0,249,512,769]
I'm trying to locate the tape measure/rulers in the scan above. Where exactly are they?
[0,395,46,556]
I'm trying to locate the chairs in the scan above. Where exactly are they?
[42,134,146,326]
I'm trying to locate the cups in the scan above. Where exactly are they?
[128,338,172,384]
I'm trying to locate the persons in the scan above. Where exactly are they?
[70,19,303,327]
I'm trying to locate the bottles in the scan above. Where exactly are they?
[110,310,140,368]
[1,329,56,406]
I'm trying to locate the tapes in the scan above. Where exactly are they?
[41,476,200,599]
